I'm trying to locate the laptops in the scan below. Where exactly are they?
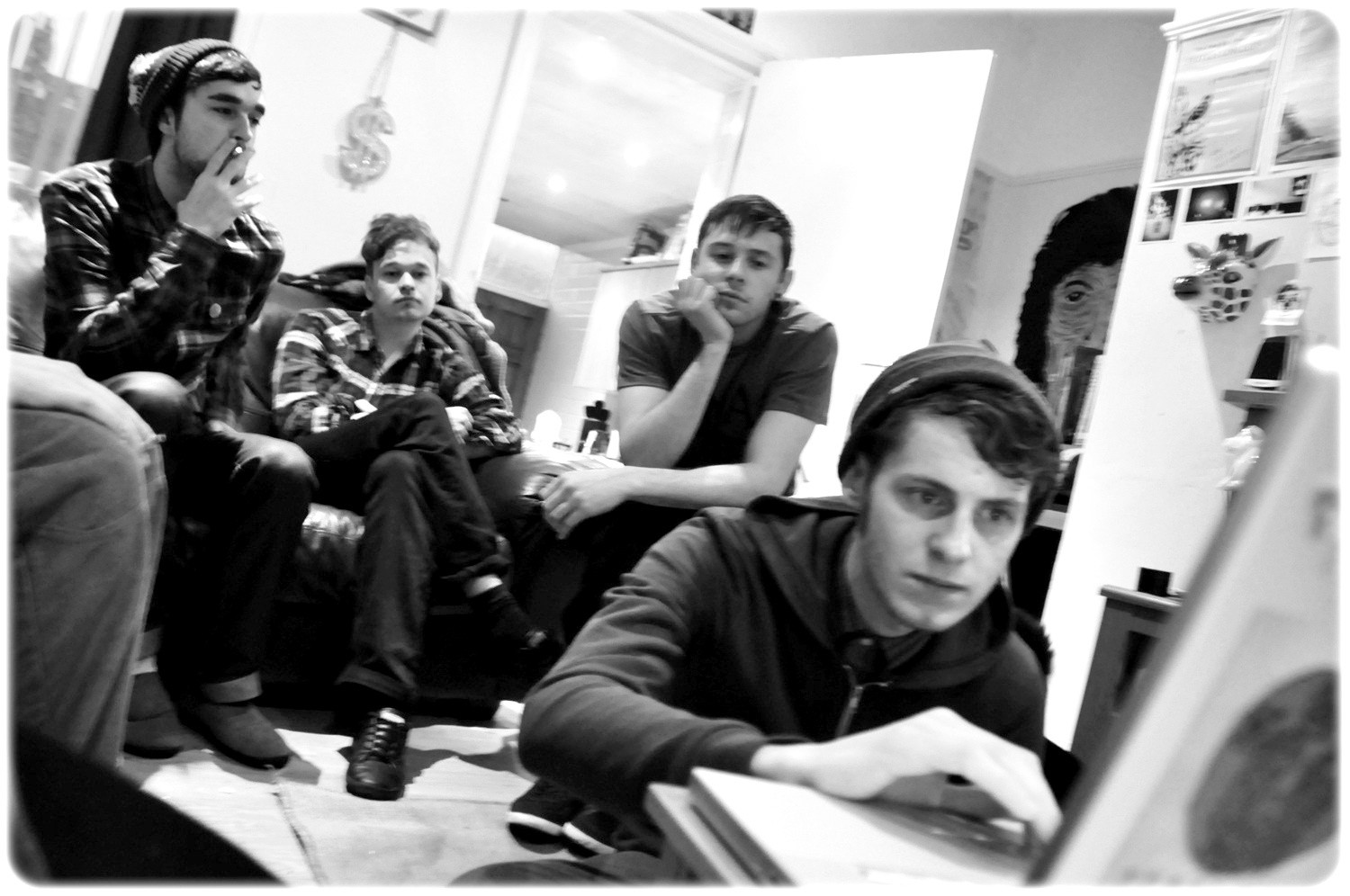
[687,345,1340,882]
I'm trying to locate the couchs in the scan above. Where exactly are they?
[8,209,511,731]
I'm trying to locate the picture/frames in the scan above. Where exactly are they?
[361,6,447,36]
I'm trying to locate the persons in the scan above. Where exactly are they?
[525,341,1064,845]
[35,37,320,772]
[270,212,546,802]
[501,193,839,860]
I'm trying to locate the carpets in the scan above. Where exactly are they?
[277,788,591,889]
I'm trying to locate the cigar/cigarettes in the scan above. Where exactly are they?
[232,146,243,156]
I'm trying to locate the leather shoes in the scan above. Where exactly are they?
[348,712,409,801]
[461,597,564,678]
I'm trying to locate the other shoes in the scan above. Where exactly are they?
[195,701,292,772]
[121,672,189,760]
[559,805,623,854]
[511,777,582,849]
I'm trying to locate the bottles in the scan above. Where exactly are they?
[577,401,609,453]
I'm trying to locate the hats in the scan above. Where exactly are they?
[128,38,246,134]
[840,342,1055,533]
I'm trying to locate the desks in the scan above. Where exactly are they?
[643,765,1036,888]
[1067,586,1186,765]
